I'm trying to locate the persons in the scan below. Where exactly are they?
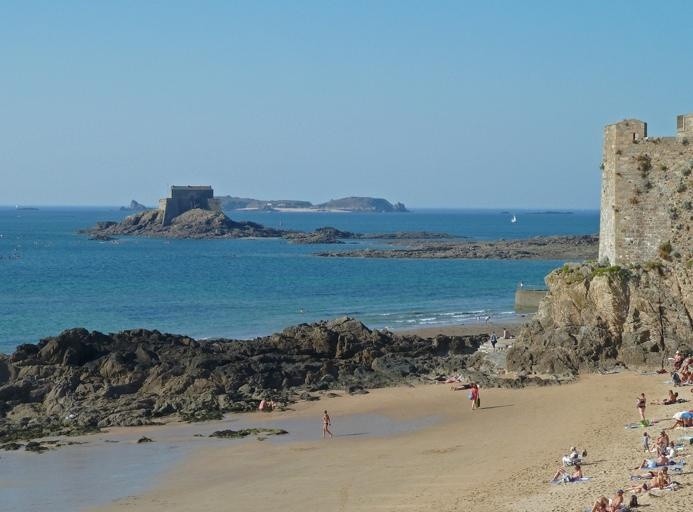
[498,327,511,339]
[258,397,267,412]
[268,397,275,410]
[468,384,479,410]
[321,409,333,439]
[489,331,497,349]
[551,464,583,482]
[590,348,692,512]
[560,445,578,467]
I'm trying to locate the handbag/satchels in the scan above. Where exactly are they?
[476,398,480,407]
[468,389,474,400]
